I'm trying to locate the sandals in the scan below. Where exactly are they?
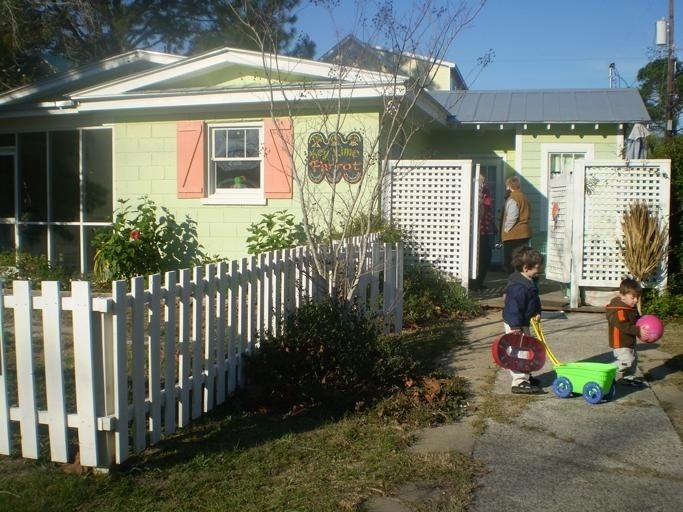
[511,381,540,395]
[529,377,541,385]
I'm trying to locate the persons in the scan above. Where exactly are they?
[500,177,532,274]
[606,278,649,386]
[502,245,542,394]
[469,174,492,291]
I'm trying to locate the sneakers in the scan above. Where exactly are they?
[621,375,647,387]
